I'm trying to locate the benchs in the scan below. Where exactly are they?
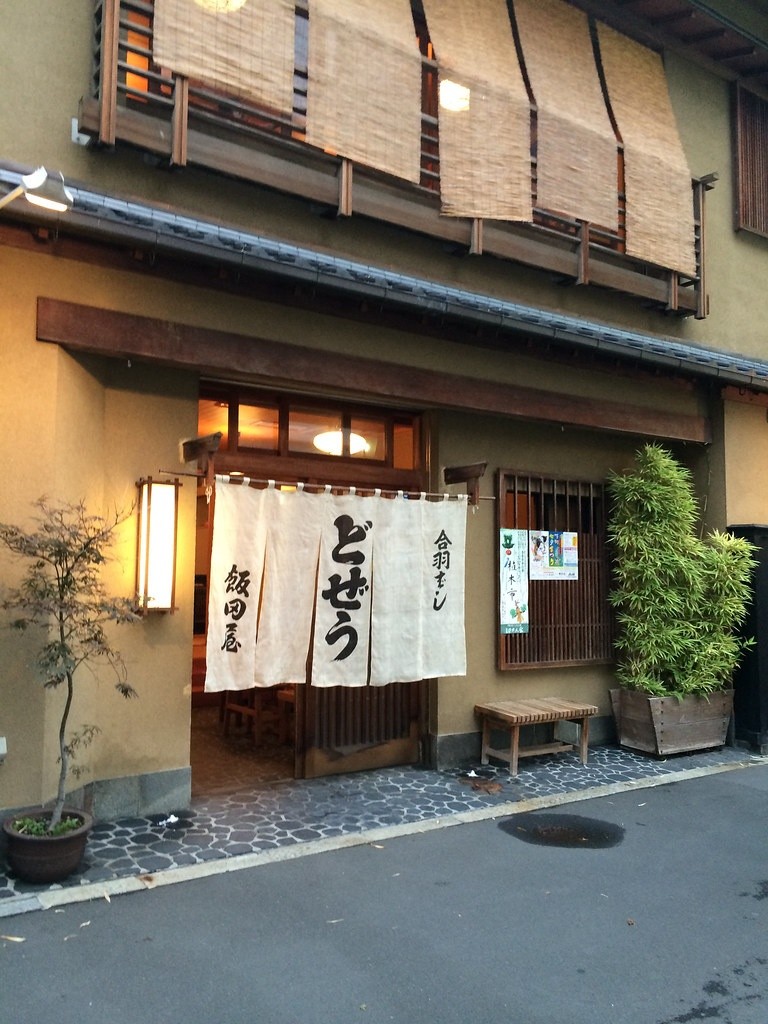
[474,695,599,775]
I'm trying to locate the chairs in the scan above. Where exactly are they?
[220,689,277,743]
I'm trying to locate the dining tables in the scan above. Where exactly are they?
[220,685,298,736]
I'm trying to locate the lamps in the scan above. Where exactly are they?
[0,166,73,216]
[132,475,183,616]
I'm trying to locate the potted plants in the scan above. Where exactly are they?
[607,441,764,758]
[1,483,153,879]
